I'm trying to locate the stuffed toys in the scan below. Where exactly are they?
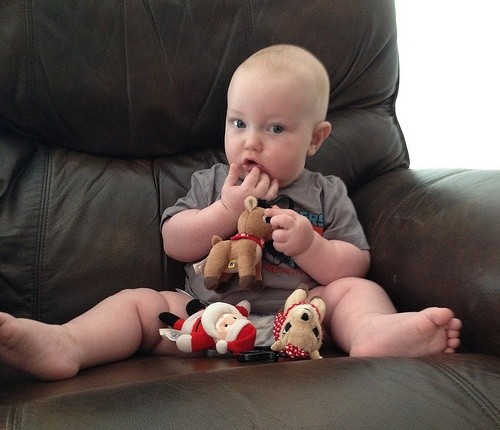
[202,196,282,292]
[158,298,258,357]
[270,282,327,361]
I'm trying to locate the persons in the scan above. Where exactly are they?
[0,41,464,380]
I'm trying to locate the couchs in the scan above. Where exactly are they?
[0,0,500,429]
